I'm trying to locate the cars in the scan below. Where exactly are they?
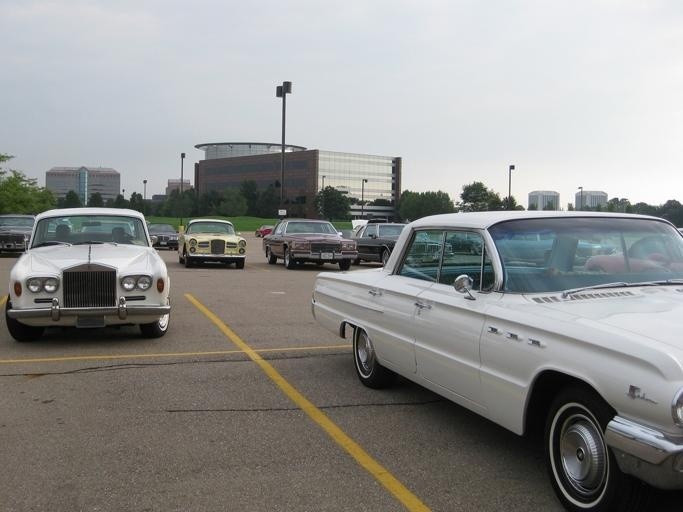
[255,224,274,238]
[146,223,179,250]
[305,208,682,511]
[583,224,682,278]
[47,218,73,241]
[4,205,171,345]
[79,220,133,239]
[177,219,247,269]
[0,214,36,254]
[493,229,616,270]
[261,218,359,271]
[446,232,484,256]
[349,217,453,267]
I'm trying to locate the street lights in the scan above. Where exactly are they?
[275,81,292,220]
[578,186,582,208]
[361,179,367,219]
[143,180,146,216]
[122,190,124,199]
[321,176,326,190]
[180,153,185,194]
[508,165,514,208]
